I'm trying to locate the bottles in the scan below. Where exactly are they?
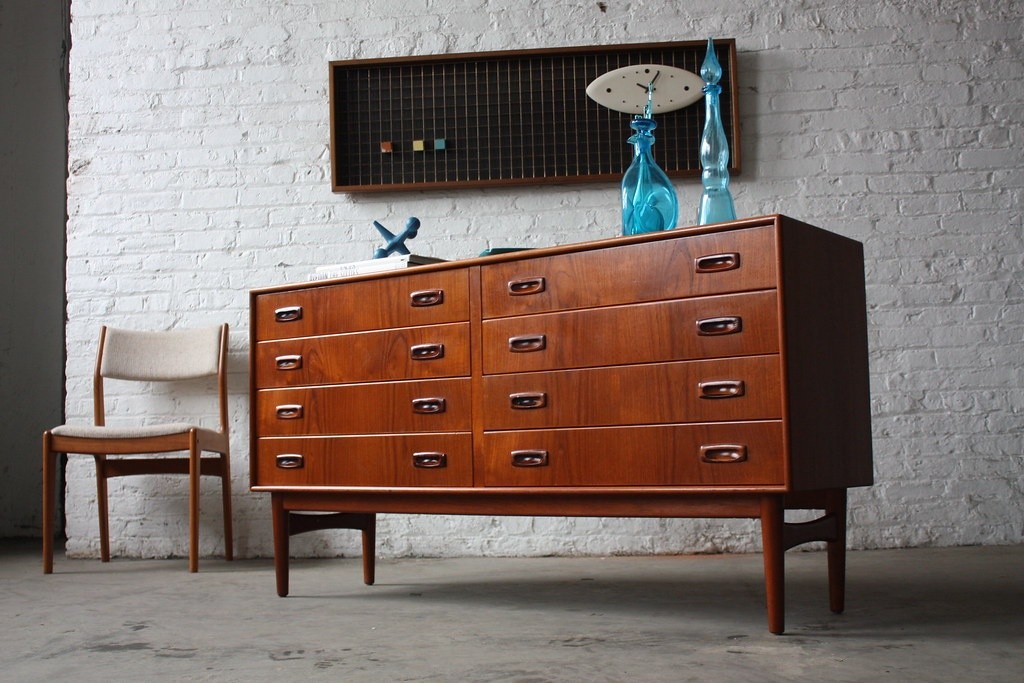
[697,37,736,226]
[620,119,679,236]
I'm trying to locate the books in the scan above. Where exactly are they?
[307,254,448,281]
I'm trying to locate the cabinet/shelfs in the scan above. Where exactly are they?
[248,215,874,636]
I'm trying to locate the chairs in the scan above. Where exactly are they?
[42,323,233,576]
